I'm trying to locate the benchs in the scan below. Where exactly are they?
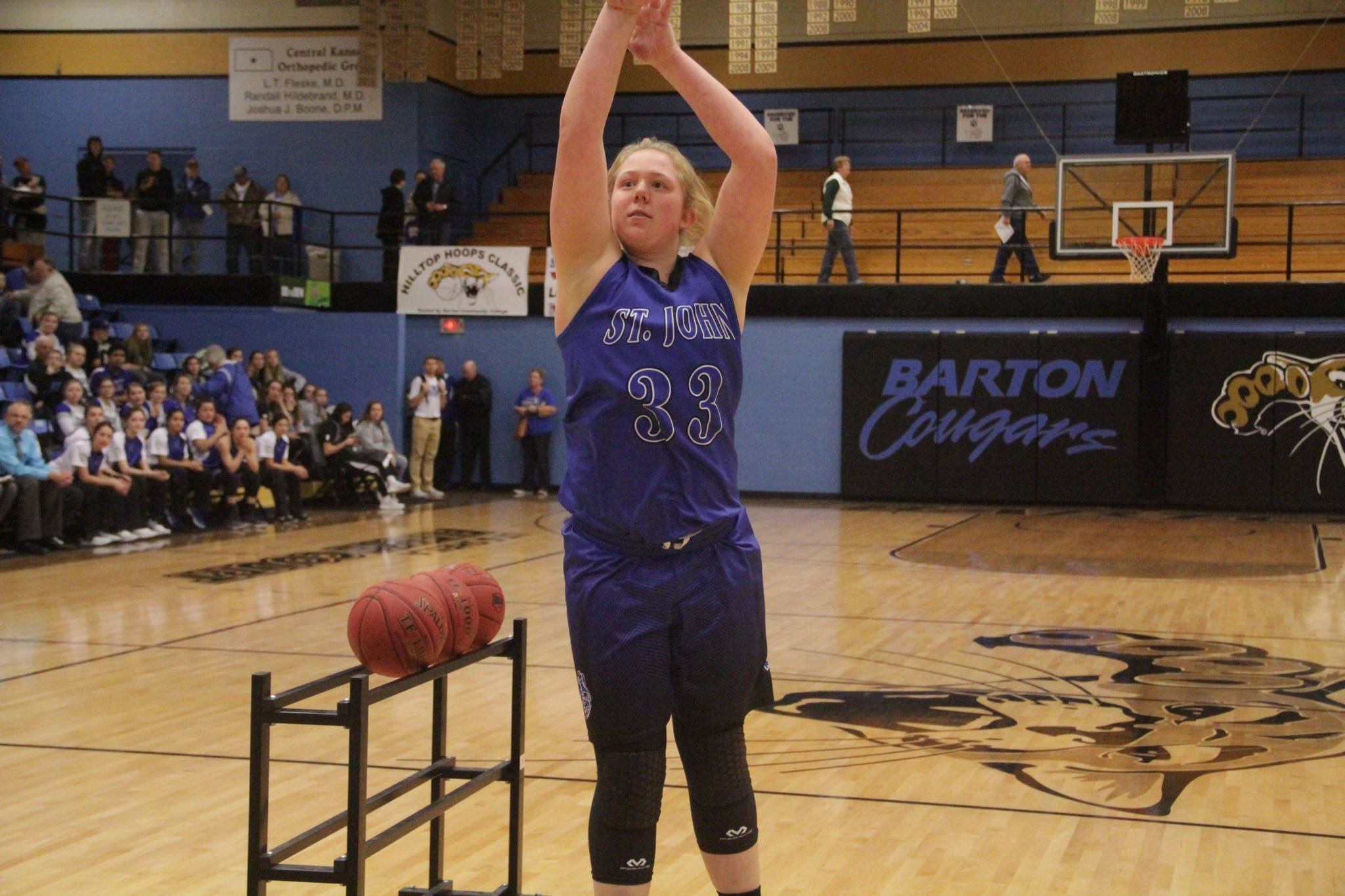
[461,158,1345,283]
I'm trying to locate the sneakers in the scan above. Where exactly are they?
[990,278,1010,284]
[410,490,431,501]
[378,496,405,510]
[168,504,186,531]
[184,508,209,530]
[0,536,64,558]
[1029,272,1052,285]
[80,522,171,548]
[387,476,412,493]
[225,509,298,529]
[425,488,445,500]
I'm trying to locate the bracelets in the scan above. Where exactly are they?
[535,405,539,415]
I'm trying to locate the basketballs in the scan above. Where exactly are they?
[434,563,506,657]
[347,578,448,678]
[404,570,479,665]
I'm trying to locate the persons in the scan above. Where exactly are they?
[512,368,558,499]
[0,239,412,554]
[550,0,777,896]
[408,357,492,500]
[817,157,869,284]
[0,136,303,277]
[376,157,466,313]
[988,154,1052,285]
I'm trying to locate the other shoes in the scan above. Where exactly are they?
[538,490,545,496]
[850,280,867,285]
[512,488,532,494]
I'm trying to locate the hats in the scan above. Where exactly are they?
[185,158,199,166]
[234,165,248,177]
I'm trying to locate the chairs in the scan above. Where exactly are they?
[0,268,198,459]
